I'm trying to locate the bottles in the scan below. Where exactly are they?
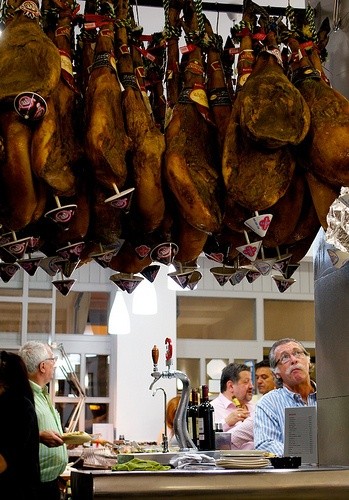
[185,385,215,451]
[232,395,248,421]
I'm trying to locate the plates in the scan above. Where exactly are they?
[58,432,99,445]
[215,453,271,470]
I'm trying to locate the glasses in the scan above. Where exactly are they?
[274,350,307,368]
[44,356,58,363]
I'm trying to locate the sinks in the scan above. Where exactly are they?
[134,452,221,466]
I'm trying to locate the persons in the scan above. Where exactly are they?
[16,340,84,500]
[208,363,262,451]
[0,349,41,500]
[249,337,318,457]
[255,360,278,395]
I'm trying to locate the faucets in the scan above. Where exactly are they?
[151,387,170,453]
[148,337,199,453]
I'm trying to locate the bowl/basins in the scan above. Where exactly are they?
[270,457,302,469]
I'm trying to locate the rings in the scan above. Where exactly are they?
[237,413,241,418]
